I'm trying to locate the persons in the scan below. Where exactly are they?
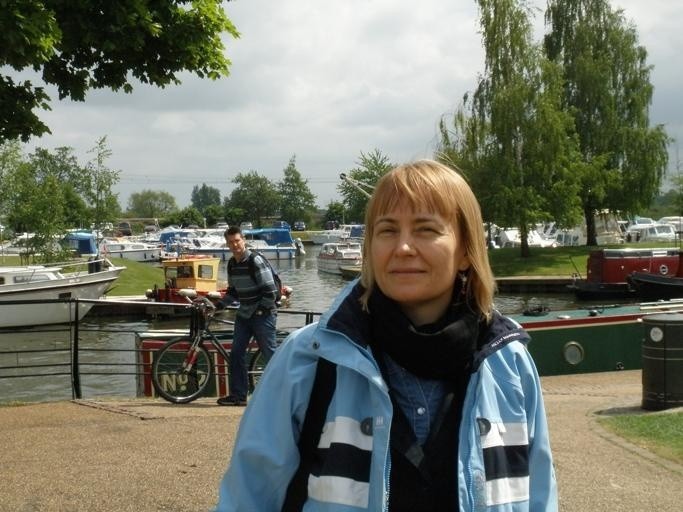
[214,227,277,406]
[212,159,559,512]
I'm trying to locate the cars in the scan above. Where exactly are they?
[90,219,341,235]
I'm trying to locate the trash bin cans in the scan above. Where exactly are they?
[88,256,96,274]
[639,313,683,411]
[95,255,104,272]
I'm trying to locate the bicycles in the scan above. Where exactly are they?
[151,289,291,405]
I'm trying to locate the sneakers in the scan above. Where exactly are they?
[217,396,248,406]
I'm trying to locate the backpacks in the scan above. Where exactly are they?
[249,252,282,303]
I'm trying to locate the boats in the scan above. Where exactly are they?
[0,257,128,323]
[625,271,683,299]
[91,253,296,318]
[315,241,362,274]
[567,244,683,299]
[321,224,366,247]
[0,227,303,261]
[483,210,683,249]
